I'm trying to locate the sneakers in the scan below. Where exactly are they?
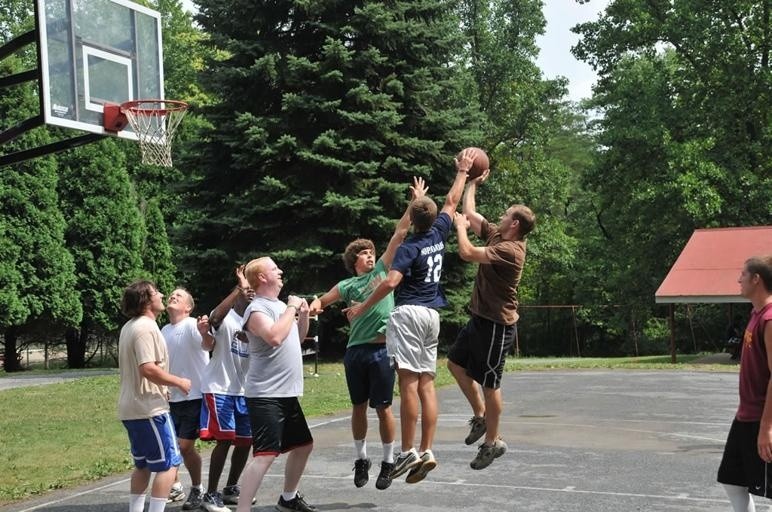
[275,491,320,512]
[470,439,508,470]
[389,449,423,479]
[375,461,395,489]
[220,484,257,505]
[183,487,207,509]
[465,411,487,445]
[166,486,186,502]
[200,493,232,511]
[352,458,372,487]
[406,448,436,483]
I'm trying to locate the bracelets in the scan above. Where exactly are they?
[287,304,298,310]
[236,285,244,291]
[458,169,469,172]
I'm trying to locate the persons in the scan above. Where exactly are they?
[118,280,192,512]
[160,286,215,510]
[447,169,535,470]
[309,176,429,490]
[199,264,257,512]
[236,256,320,512]
[727,314,743,360]
[716,258,772,512]
[341,147,477,484]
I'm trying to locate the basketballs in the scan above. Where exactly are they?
[458,147,489,180]
